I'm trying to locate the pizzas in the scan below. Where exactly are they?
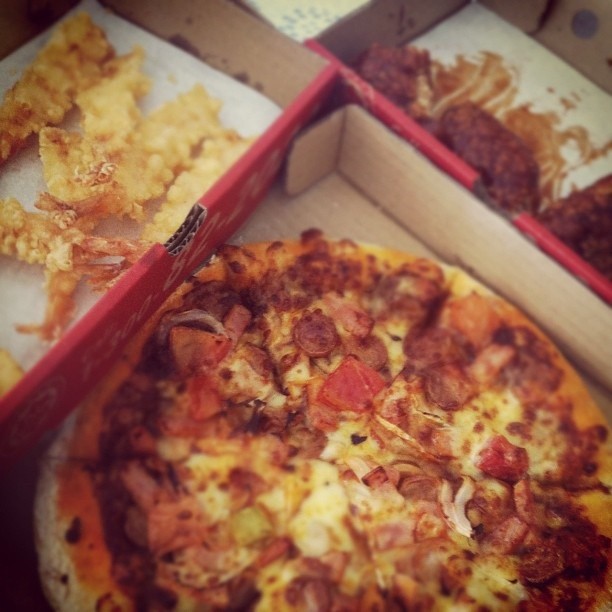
[34,229,611,607]
